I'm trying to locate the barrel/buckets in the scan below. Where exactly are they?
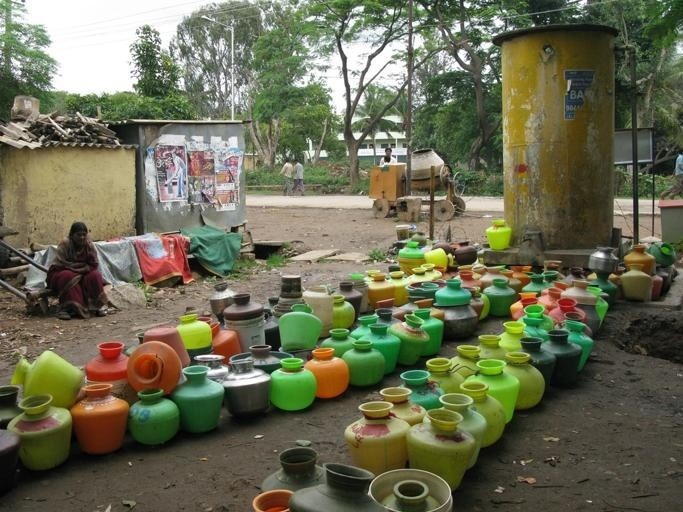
[0,219,676,512]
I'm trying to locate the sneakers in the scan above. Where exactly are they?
[59,311,71,319]
[660,194,675,200]
[96,304,108,316]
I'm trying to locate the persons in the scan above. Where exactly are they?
[383,155,391,165]
[669,150,683,200]
[46,221,109,319]
[280,159,294,195]
[290,159,304,196]
[380,148,397,166]
[660,168,683,200]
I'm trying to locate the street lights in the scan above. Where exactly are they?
[198,13,237,121]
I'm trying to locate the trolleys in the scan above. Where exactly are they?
[0,235,108,315]
